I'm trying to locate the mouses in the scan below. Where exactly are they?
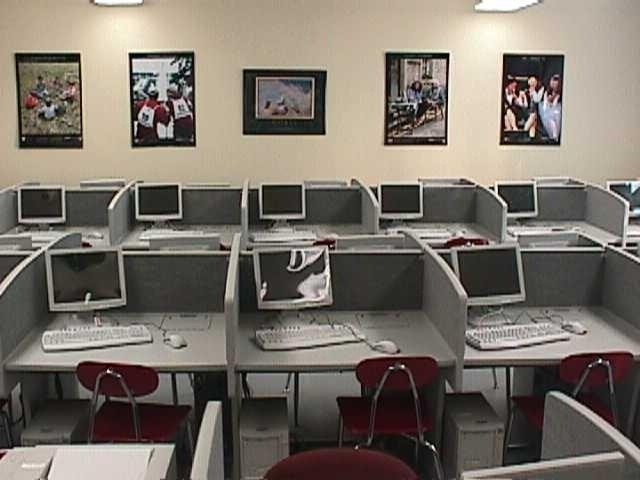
[163,334,187,349]
[372,340,401,354]
[563,321,588,336]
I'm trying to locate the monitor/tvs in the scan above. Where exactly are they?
[253,246,334,327]
[45,246,129,326]
[454,245,528,327]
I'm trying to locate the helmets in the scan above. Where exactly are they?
[149,88,159,99]
[45,97,52,107]
[166,88,177,98]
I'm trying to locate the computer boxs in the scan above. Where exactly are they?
[17,395,91,446]
[240,397,290,480]
[441,390,507,480]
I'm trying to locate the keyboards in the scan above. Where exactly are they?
[256,322,366,351]
[40,323,153,351]
[465,322,568,349]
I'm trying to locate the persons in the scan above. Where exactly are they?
[503,77,528,133]
[260,86,311,117]
[163,79,195,142]
[132,88,171,142]
[22,69,80,120]
[521,75,548,137]
[537,75,560,139]
[421,77,445,116]
[410,80,428,127]
